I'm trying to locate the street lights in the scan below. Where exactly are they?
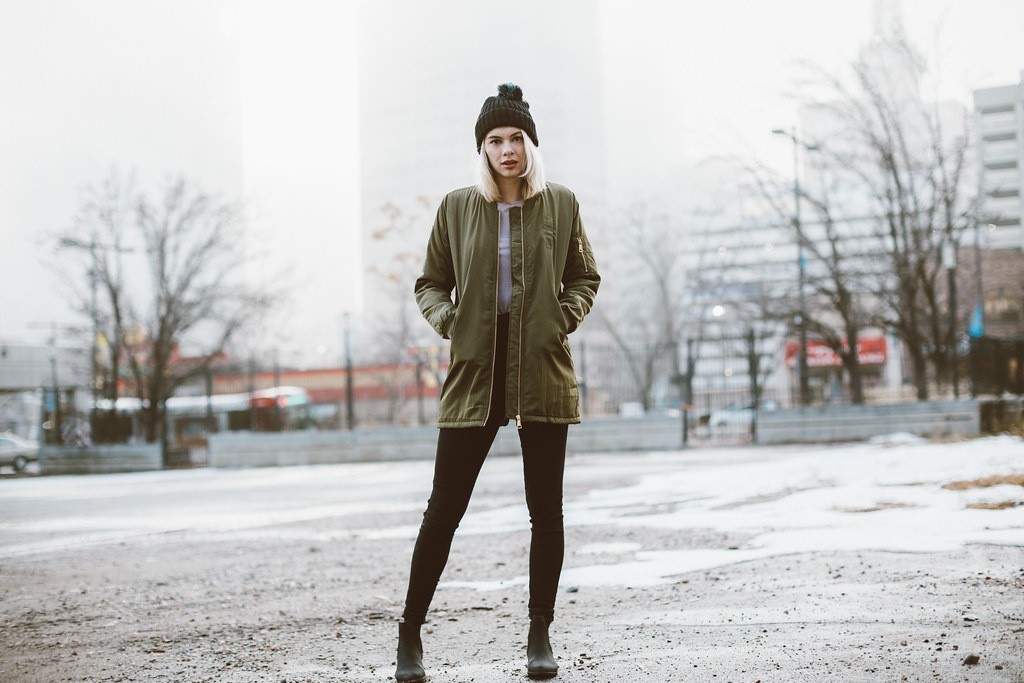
[772,127,819,405]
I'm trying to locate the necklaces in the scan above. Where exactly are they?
[502,200,510,204]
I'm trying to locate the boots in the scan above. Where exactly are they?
[527,621,558,676]
[395,622,426,683]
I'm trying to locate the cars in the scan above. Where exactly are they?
[1,432,40,473]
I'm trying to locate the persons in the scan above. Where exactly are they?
[394,85,602,683]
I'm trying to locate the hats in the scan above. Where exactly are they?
[475,83,539,155]
